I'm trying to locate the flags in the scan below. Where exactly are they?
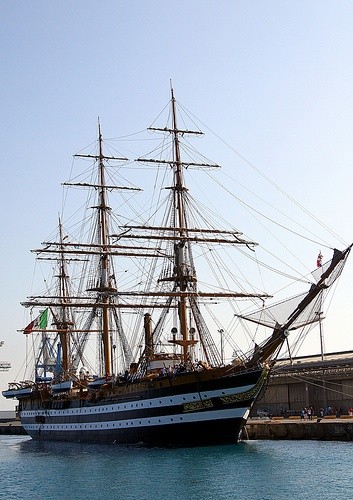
[23,307,48,334]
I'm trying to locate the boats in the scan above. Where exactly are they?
[13,385,33,398]
[87,374,106,389]
[1,388,17,399]
[50,380,74,392]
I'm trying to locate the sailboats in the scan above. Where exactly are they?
[0,77,353,448]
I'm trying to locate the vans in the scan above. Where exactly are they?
[257,407,273,417]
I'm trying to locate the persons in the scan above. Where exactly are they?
[125,368,129,375]
[300,407,313,421]
[328,404,338,417]
[163,362,184,376]
[348,407,353,415]
